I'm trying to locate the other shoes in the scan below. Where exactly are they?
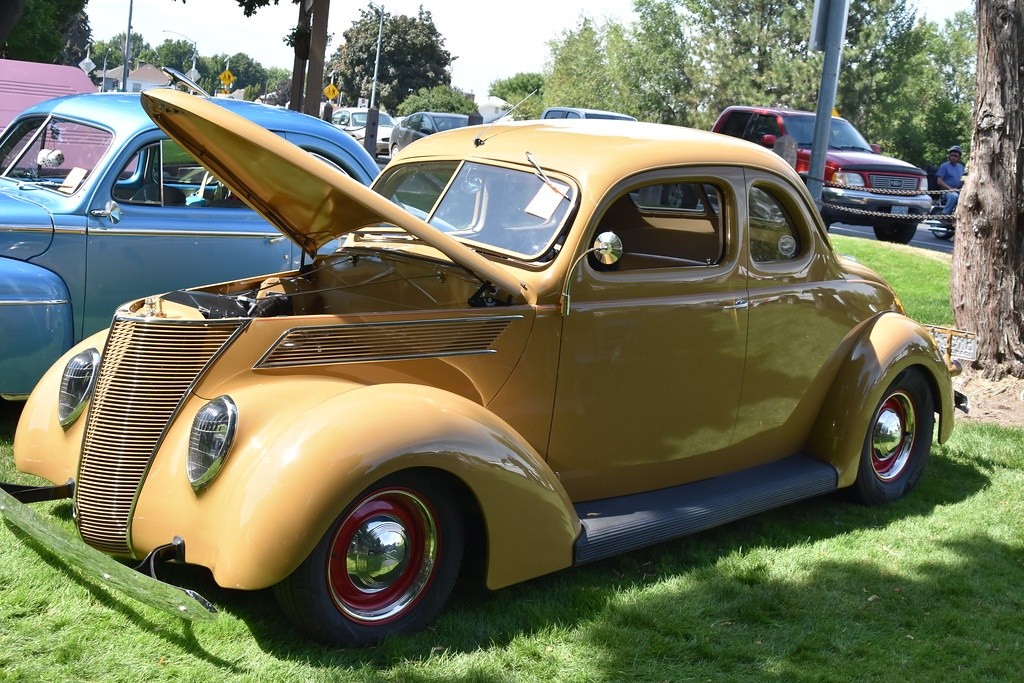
[939,219,952,226]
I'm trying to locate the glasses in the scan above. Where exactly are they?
[950,154,960,157]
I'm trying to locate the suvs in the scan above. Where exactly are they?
[710,106,932,244]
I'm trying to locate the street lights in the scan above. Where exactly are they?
[160,28,201,95]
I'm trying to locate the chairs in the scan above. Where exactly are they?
[127,182,186,207]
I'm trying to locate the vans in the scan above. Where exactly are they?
[539,104,640,131]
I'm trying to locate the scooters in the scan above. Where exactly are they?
[924,198,961,239]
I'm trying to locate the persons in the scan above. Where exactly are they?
[935,146,966,225]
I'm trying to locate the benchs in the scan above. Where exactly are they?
[613,229,792,275]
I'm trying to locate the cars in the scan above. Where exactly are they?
[388,112,519,199]
[327,103,399,162]
[0,90,478,410]
[7,68,972,647]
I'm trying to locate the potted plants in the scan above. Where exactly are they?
[286,21,329,61]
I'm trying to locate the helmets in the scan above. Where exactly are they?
[949,145,962,155]
